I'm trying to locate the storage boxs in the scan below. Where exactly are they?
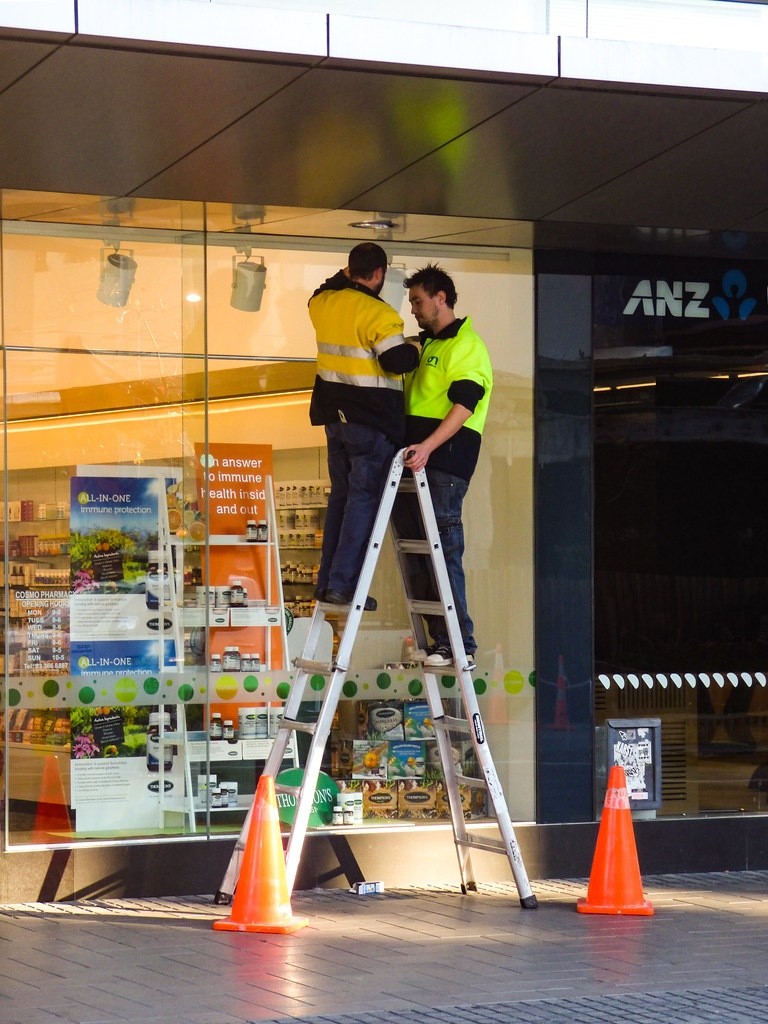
[327,660,488,824]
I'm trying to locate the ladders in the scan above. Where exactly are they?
[213,448,541,915]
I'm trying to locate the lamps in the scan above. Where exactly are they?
[230,204,270,311]
[96,195,138,308]
[371,229,406,316]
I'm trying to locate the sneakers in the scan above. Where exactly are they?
[411,645,438,660]
[315,587,327,601]
[327,588,378,611]
[423,645,473,667]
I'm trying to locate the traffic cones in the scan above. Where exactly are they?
[31,755,74,845]
[576,766,655,916]
[212,775,310,935]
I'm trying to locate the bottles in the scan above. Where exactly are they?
[210,707,284,740]
[183,565,248,610]
[274,507,324,619]
[0,534,71,561]
[146,712,174,772]
[406,638,414,663]
[211,784,229,808]
[210,646,261,672]
[245,520,269,543]
[145,549,175,609]
[0,565,71,655]
[331,791,363,826]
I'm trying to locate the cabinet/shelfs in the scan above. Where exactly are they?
[0,503,328,806]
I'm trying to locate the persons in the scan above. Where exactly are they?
[308,243,422,611]
[402,265,495,668]
